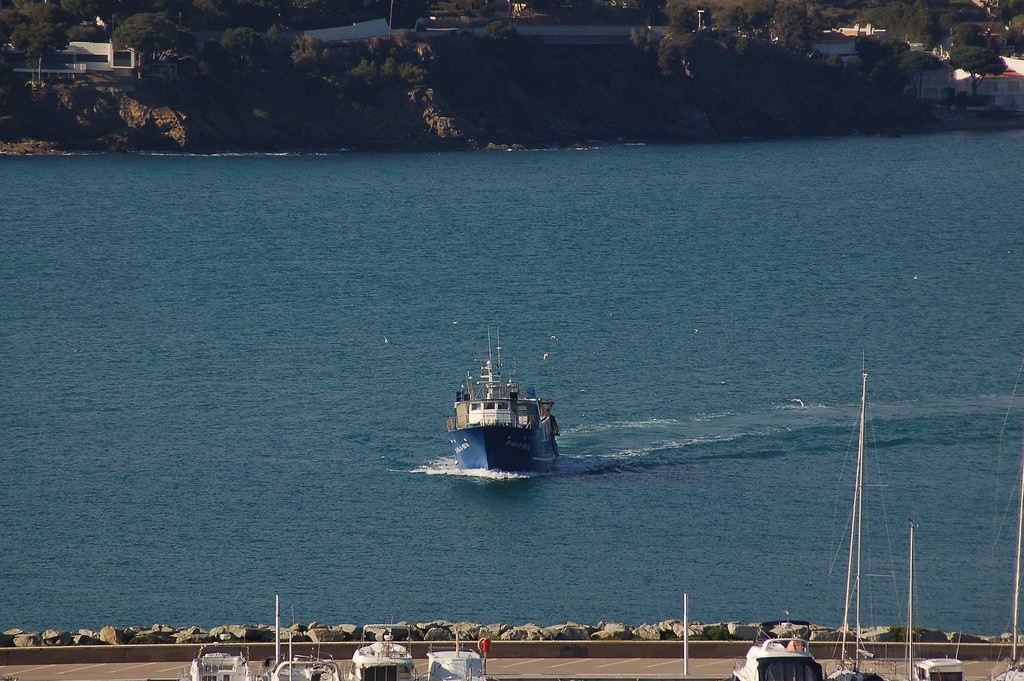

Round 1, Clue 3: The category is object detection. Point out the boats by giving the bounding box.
[255,594,341,681]
[342,623,418,681]
[176,643,255,681]
[415,630,492,681]
[725,366,1024,681]
[445,324,561,472]
